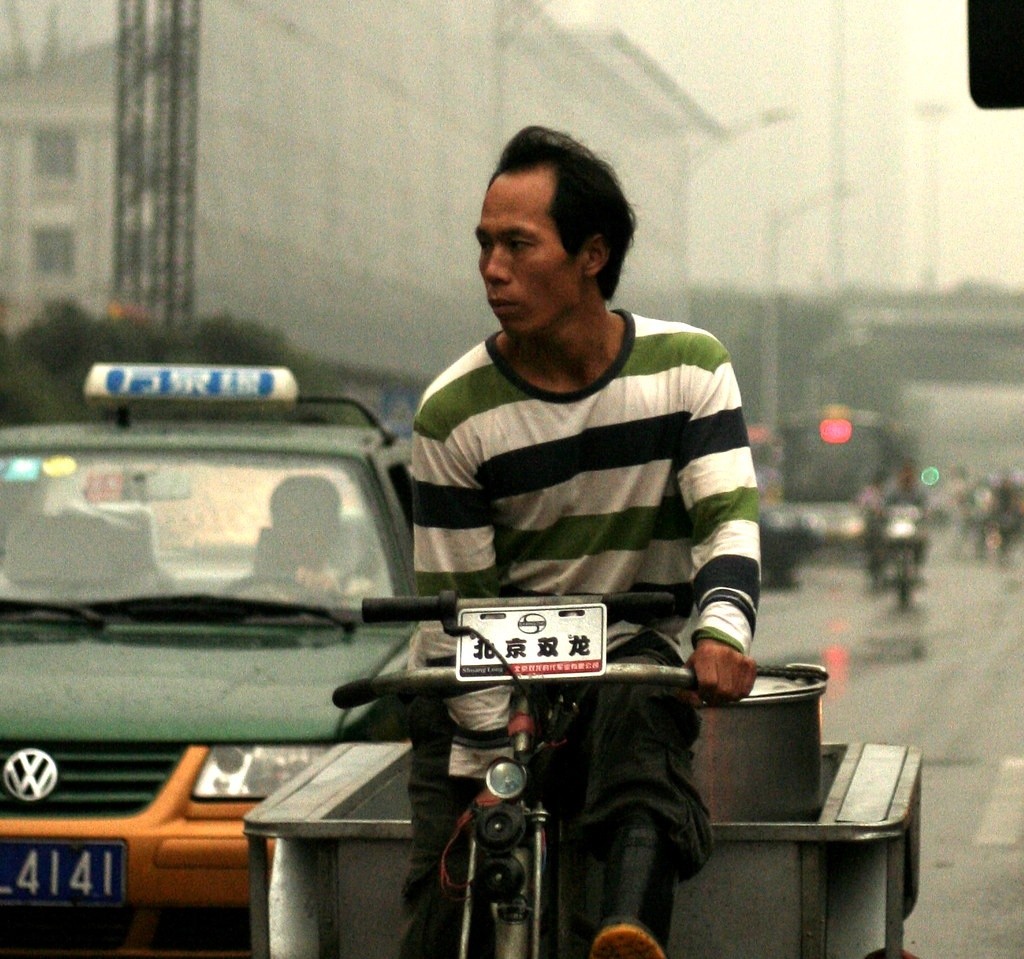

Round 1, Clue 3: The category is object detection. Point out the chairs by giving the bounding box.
[49,505,152,596]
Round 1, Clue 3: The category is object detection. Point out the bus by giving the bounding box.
[773,405,903,554]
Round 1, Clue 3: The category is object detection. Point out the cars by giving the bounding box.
[0,364,419,909]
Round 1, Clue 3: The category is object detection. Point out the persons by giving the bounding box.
[862,457,1024,596]
[236,476,379,602]
[396,126,761,959]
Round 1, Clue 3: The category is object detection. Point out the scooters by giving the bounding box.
[870,498,926,608]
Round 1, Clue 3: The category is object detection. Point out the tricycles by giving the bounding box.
[246,587,920,959]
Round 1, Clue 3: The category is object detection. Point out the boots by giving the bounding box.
[589,823,668,959]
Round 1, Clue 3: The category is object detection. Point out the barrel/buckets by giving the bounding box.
[691,663,829,823]
